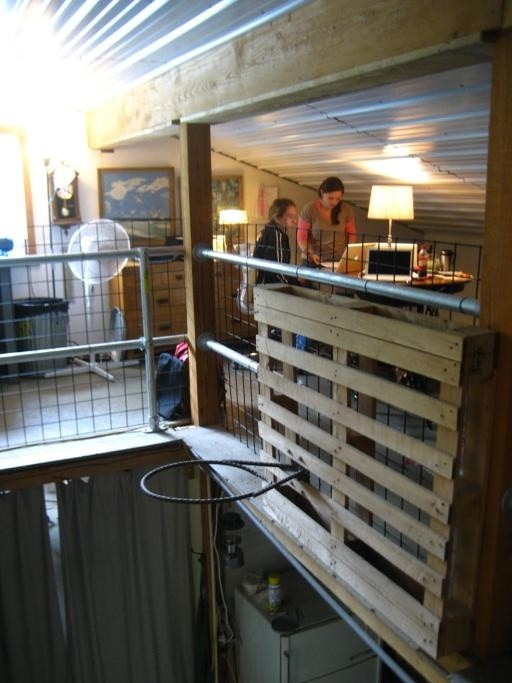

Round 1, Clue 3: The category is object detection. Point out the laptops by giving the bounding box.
[364,249,413,282]
[325,242,379,274]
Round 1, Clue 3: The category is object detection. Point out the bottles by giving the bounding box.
[439,249,455,271]
[418,244,429,279]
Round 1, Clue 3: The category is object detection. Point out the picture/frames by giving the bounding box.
[212,175,245,243]
[98,166,176,247]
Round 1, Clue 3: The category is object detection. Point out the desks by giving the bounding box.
[319,266,470,295]
[0,257,41,383]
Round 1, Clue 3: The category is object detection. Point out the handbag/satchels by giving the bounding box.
[156,352,226,421]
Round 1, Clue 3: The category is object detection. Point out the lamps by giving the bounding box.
[366,184,414,246]
[220,209,249,253]
[219,511,245,569]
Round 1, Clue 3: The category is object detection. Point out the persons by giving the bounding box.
[296,176,358,285]
[252,197,310,375]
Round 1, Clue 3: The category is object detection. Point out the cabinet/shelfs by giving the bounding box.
[108,257,257,359]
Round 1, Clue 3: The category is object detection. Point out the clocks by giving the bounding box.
[47,162,81,235]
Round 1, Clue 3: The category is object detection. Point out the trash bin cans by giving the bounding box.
[14,297,69,378]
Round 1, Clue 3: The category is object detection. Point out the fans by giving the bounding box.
[44,218,143,382]
